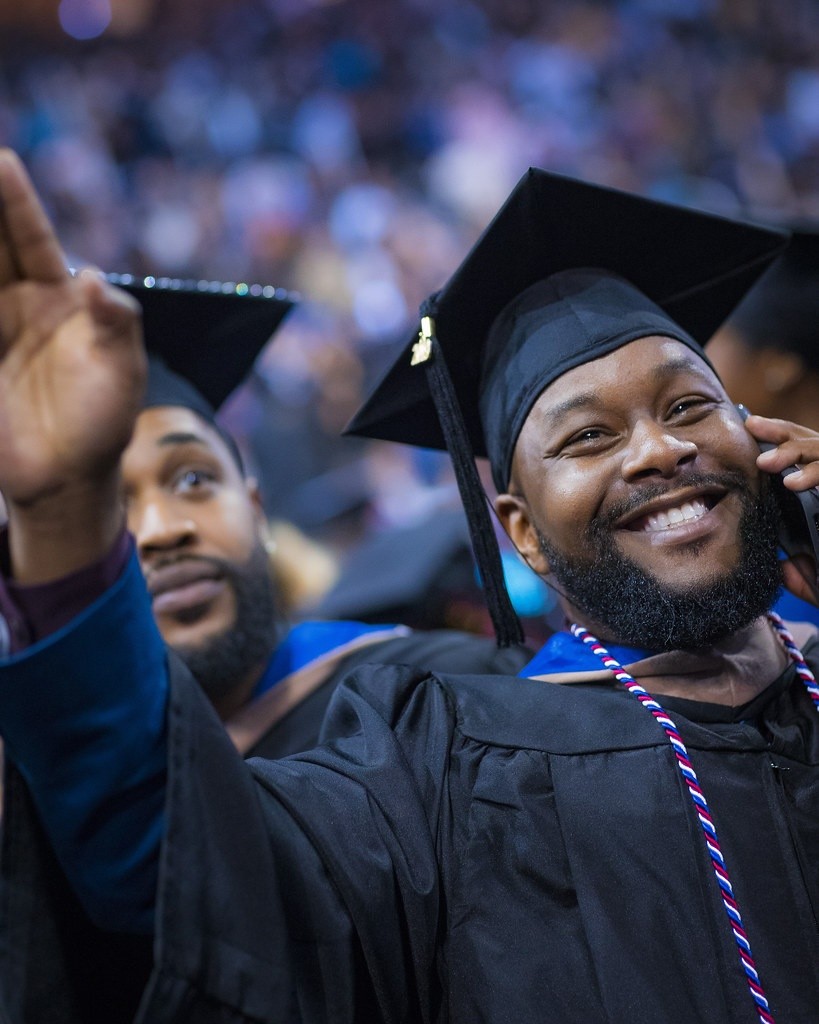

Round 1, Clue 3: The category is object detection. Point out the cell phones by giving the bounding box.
[733,401,819,598]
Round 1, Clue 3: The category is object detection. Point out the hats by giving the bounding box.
[70,270,302,422]
[342,167,793,651]
[304,494,473,632]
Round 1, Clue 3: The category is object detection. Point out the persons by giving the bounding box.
[272,467,550,649]
[0,146,819,1024]
[114,269,415,755]
[701,216,819,606]
